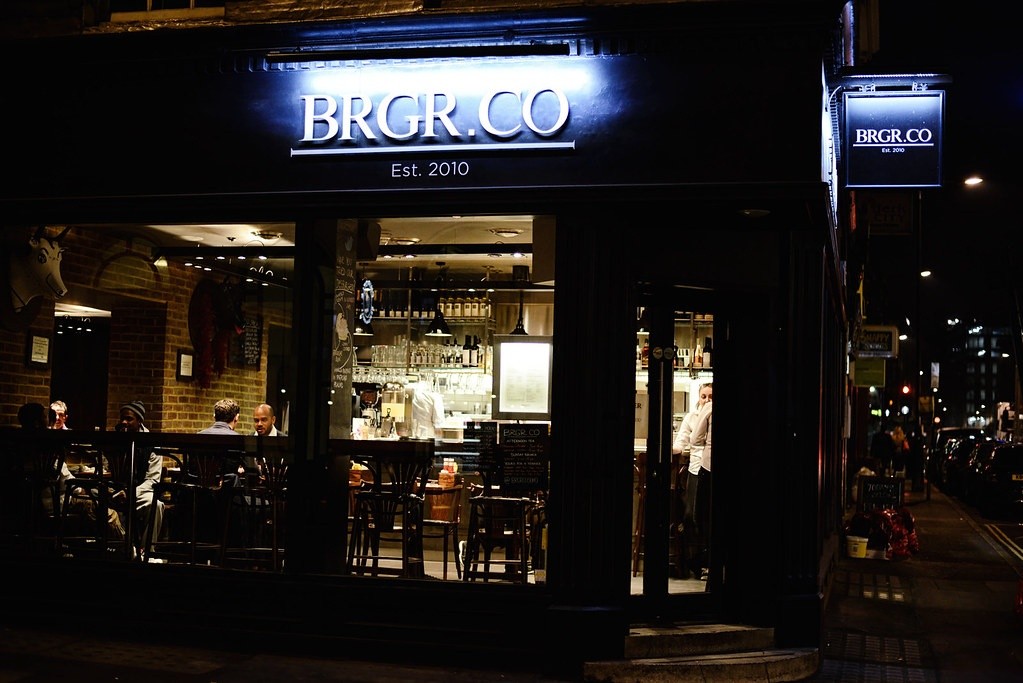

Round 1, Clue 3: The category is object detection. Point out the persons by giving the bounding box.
[673,381,712,579]
[245,404,288,480]
[16,403,125,540]
[49,401,107,473]
[188,399,257,474]
[689,402,712,581]
[405,370,444,438]
[871,423,910,474]
[102,401,164,550]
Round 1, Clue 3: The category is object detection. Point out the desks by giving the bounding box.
[364,480,462,494]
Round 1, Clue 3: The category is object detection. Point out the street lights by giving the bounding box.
[898,174,985,494]
[901,405,910,434]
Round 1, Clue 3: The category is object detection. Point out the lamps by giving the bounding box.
[425,293,453,337]
[489,228,519,237]
[352,262,374,336]
[506,291,529,334]
[391,237,420,245]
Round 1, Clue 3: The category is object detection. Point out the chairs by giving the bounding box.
[0,438,546,588]
[631,450,691,577]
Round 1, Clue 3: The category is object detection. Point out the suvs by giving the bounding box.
[936,427,986,458]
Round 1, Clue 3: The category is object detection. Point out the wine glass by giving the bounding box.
[352,346,358,366]
[352,368,408,384]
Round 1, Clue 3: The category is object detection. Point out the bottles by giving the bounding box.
[371,333,482,368]
[356,289,491,318]
[442,458,456,473]
[636,336,713,370]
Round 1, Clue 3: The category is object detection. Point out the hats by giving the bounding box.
[120,399,145,423]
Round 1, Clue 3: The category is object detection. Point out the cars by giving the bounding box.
[947,439,1023,510]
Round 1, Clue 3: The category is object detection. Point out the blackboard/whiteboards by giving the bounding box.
[331,350,354,426]
[460,422,498,473]
[499,424,550,497]
[227,312,262,372]
[857,476,904,515]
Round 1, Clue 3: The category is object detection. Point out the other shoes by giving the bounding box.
[668,567,691,580]
[701,567,709,581]
